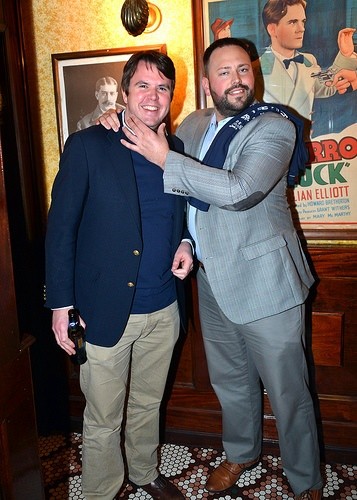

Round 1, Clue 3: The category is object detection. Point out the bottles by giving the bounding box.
[67,309,87,365]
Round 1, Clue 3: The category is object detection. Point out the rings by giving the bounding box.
[190,268,193,271]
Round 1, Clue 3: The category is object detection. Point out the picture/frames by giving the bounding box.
[191,0,357,239]
[52,43,172,154]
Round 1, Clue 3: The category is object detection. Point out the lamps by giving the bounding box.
[120,0,162,37]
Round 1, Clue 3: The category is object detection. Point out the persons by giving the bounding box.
[45,51,194,499]
[76,76,125,131]
[95,38,322,500]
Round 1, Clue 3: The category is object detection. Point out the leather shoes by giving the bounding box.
[296,488,319,500]
[141,475,186,500]
[206,456,260,492]
